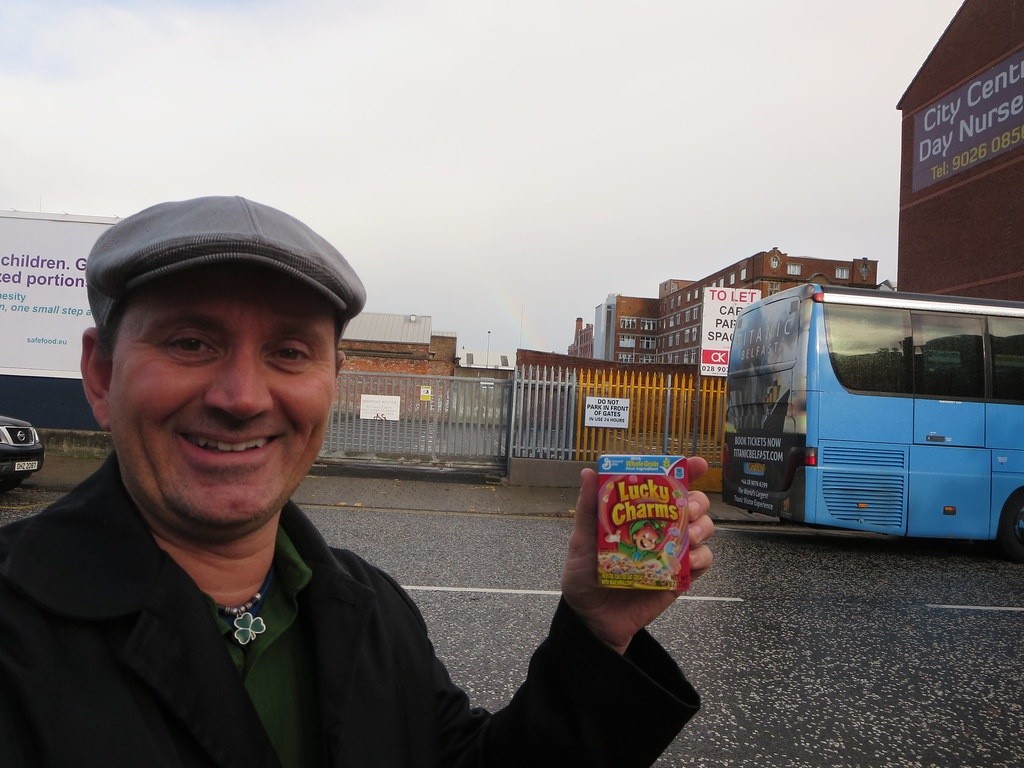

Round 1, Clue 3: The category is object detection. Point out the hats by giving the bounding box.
[84,195,366,343]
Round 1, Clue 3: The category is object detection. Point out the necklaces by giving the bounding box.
[213,567,274,645]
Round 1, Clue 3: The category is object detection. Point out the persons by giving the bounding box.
[0,196,715,768]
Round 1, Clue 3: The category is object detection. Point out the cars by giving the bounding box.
[0,414,44,491]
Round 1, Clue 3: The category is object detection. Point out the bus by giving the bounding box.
[721,283,1024,558]
